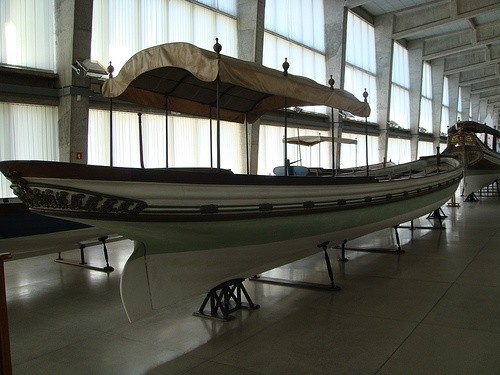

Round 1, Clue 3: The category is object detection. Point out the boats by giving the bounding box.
[418,119,499,203]
[0,33,466,324]
